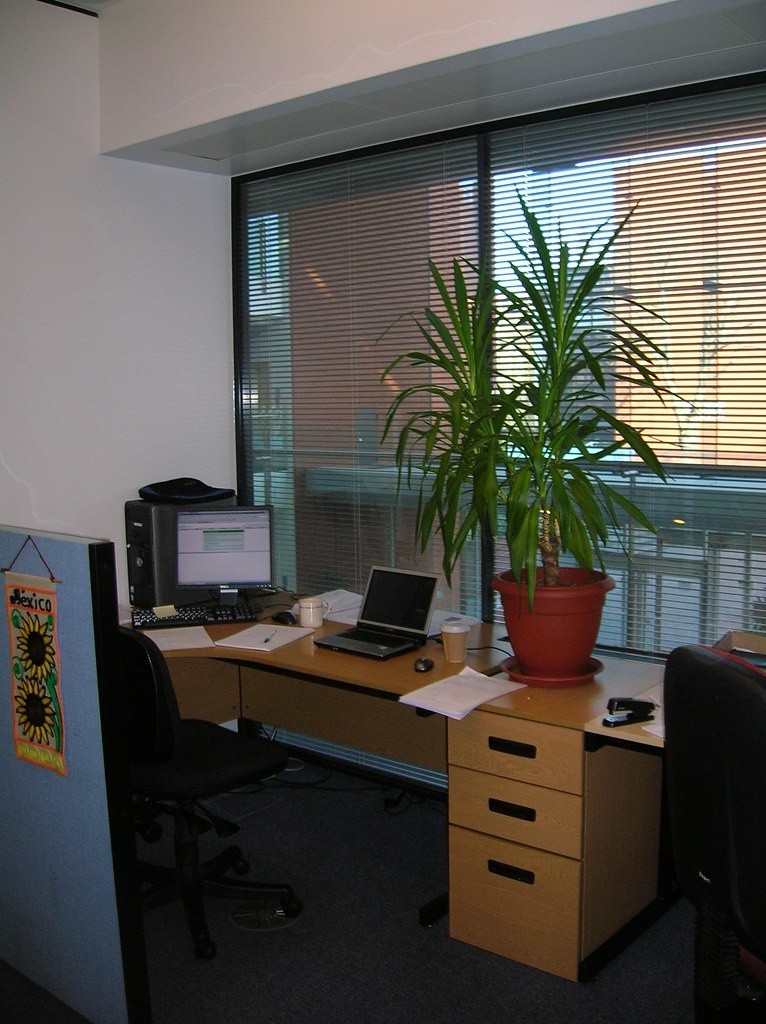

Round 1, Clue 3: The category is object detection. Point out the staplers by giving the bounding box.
[603,697,655,728]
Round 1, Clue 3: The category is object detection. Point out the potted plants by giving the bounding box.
[373,183,696,690]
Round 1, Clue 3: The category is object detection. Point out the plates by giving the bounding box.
[500,656,605,686]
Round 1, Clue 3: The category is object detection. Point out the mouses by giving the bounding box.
[272,611,297,625]
[415,657,434,673]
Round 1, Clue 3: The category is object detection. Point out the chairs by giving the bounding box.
[118,626,304,961]
[663,643,766,1024]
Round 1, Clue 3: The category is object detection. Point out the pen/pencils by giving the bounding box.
[265,630,278,644]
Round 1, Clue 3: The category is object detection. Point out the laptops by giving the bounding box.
[311,566,443,660]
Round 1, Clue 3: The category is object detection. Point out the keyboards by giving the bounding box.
[132,606,259,630]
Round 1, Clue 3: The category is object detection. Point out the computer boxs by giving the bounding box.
[125,490,247,611]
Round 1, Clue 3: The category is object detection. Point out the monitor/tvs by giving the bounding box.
[174,506,274,607]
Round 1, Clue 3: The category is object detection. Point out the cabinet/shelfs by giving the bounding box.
[122,590,666,985]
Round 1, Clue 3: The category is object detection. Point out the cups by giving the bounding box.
[298,598,330,628]
[441,621,470,663]
